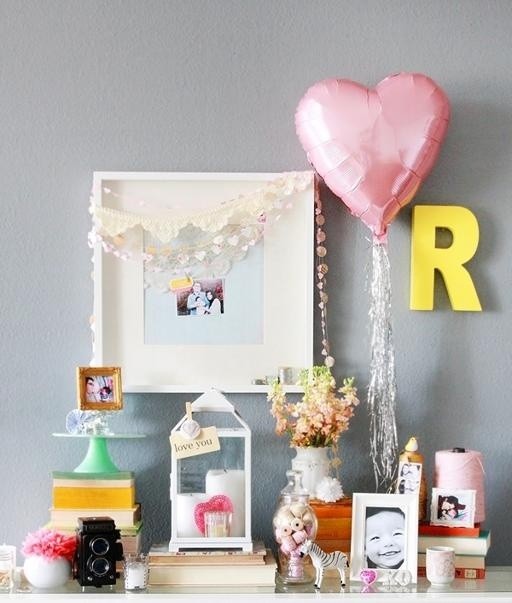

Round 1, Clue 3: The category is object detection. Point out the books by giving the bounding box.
[47,466,278,585]
[417,519,492,579]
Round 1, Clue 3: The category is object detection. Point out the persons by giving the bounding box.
[99,386,110,401]
[397,463,420,493]
[195,295,205,314]
[436,494,458,518]
[84,375,99,402]
[186,281,210,315]
[203,288,221,313]
[365,506,407,569]
[440,508,456,518]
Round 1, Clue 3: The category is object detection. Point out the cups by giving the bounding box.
[203,510,233,539]
[122,552,150,591]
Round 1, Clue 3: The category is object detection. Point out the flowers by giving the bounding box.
[20,527,77,566]
[266,365,361,450]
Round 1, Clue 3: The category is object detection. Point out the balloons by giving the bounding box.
[294,71,450,243]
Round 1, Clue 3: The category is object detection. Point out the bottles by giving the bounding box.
[271,467,321,586]
[397,436,428,521]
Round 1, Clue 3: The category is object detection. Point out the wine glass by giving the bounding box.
[425,546,456,593]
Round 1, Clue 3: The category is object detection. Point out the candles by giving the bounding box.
[176,468,246,538]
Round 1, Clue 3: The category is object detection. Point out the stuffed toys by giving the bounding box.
[299,536,349,588]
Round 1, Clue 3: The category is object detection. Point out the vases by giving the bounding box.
[291,447,332,500]
[22,557,71,588]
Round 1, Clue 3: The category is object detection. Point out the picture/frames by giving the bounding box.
[349,493,420,585]
[76,365,124,412]
[429,487,477,529]
[91,171,316,395]
